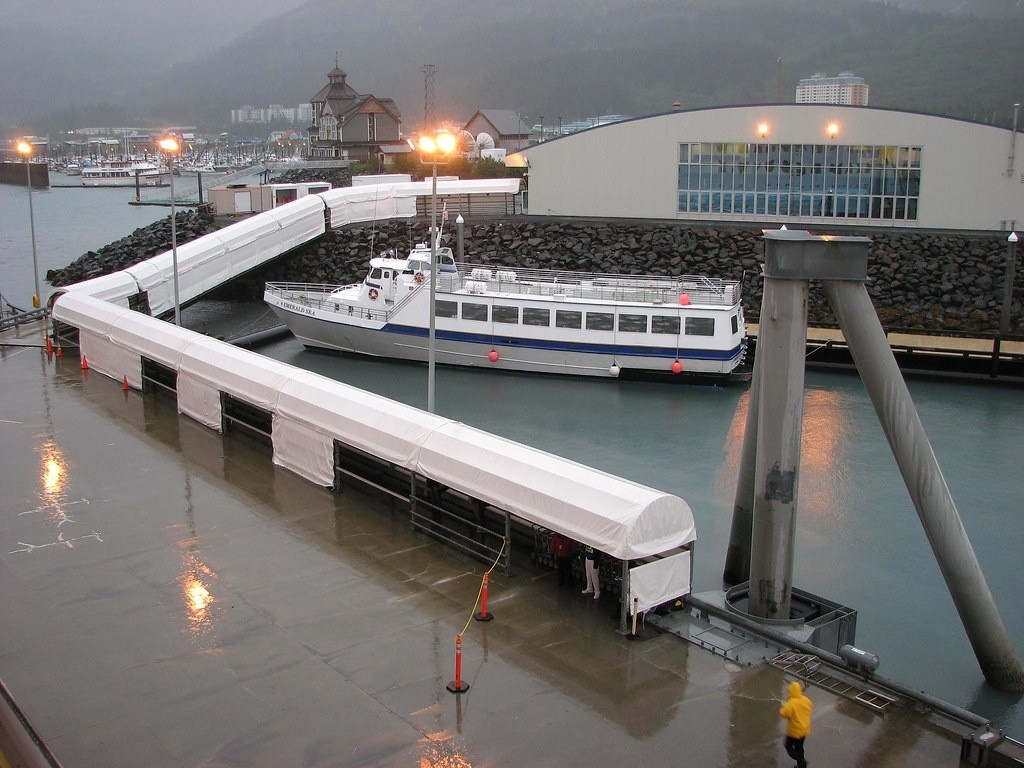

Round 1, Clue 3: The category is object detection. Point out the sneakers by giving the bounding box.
[582,589,593,593]
[594,592,600,599]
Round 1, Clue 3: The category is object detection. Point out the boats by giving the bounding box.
[264,228,749,381]
[3,150,302,188]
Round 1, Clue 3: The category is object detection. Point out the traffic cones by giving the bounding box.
[121,373,131,390]
[56,341,64,356]
[46,338,56,352]
[80,354,90,369]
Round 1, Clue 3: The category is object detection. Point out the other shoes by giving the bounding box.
[794,765,799,768]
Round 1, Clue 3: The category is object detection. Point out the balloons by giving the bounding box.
[609,366,620,375]
[489,352,499,362]
[672,362,682,373]
[679,294,690,305]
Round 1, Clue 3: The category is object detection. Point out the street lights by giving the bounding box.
[539,114,545,143]
[415,133,455,415]
[558,116,563,139]
[507,113,530,151]
[160,137,183,327]
[17,138,42,306]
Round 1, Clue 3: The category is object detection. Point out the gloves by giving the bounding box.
[593,568,599,574]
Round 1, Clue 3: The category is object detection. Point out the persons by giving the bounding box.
[780,681,813,768]
